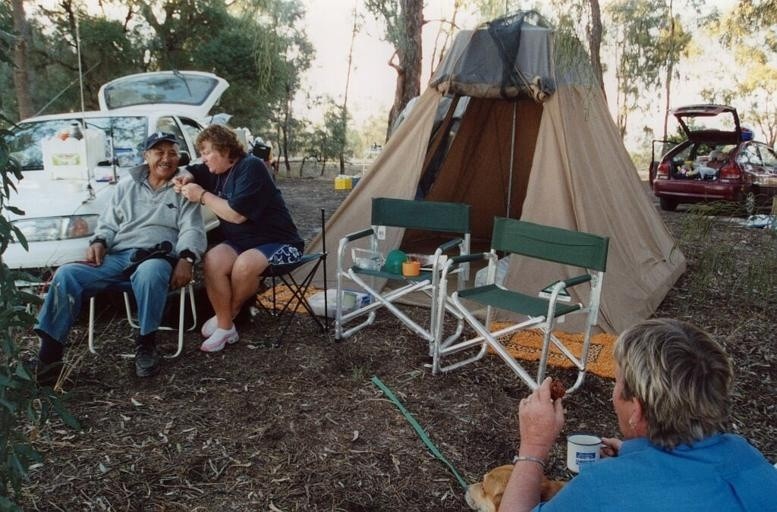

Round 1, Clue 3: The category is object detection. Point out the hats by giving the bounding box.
[145,131,180,151]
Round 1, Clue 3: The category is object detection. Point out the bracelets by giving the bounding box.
[512,455,546,468]
[200,190,207,206]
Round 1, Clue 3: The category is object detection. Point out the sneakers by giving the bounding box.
[201,314,219,339]
[135,333,159,377]
[23,357,63,384]
[200,323,239,352]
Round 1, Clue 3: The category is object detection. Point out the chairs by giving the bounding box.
[433,217,610,394]
[247,208,330,348]
[88,265,197,360]
[335,197,471,358]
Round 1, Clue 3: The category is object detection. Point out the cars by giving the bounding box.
[1,69,229,270]
[645,102,776,215]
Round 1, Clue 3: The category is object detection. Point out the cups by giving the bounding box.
[343,290,357,306]
[568,436,609,473]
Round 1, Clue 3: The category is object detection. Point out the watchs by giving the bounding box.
[182,257,193,263]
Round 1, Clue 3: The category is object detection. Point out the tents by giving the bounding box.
[283,9,686,335]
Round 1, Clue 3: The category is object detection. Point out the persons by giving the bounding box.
[32,132,207,387]
[172,124,306,353]
[495,318,777,512]
